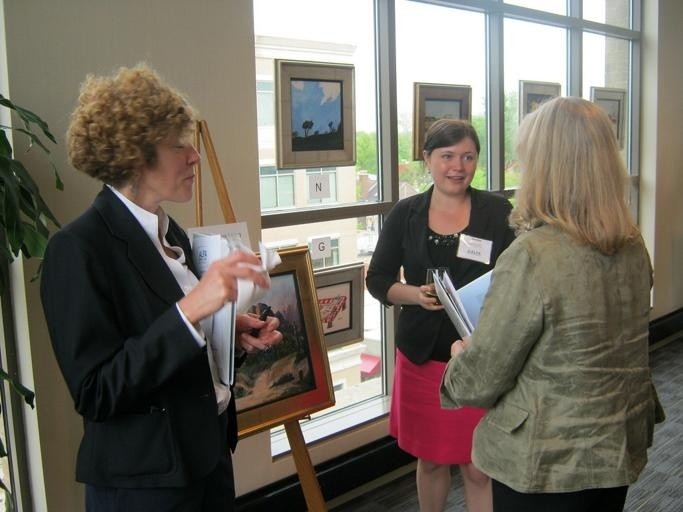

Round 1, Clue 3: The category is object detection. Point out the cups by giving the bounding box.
[424,267,451,303]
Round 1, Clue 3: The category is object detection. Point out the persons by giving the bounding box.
[438,97,666,512]
[365,118,515,512]
[41,60,285,512]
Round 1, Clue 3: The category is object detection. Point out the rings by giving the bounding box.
[264,344,273,354]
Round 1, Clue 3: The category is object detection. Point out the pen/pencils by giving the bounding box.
[235,306,271,368]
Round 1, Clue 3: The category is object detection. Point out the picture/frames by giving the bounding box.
[411,80,473,161]
[274,56,359,170]
[516,79,562,130]
[313,260,365,353]
[590,86,627,150]
[205,246,336,444]
